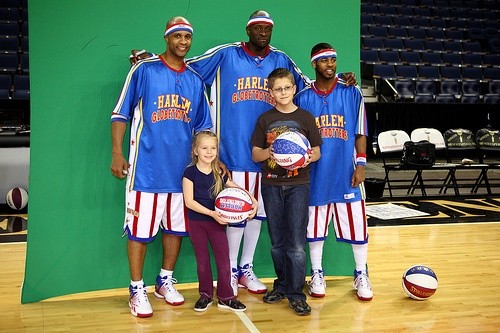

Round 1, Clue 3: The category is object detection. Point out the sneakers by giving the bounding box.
[263,289,288,303]
[194,295,213,311]
[237,265,267,294]
[154,275,185,305]
[354,272,373,301]
[128,284,153,317]
[218,299,246,311]
[230,267,238,296]
[307,270,325,297]
[289,298,311,316]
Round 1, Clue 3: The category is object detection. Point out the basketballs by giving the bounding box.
[6,186,29,210]
[215,188,252,224]
[273,130,312,171]
[402,265,438,301]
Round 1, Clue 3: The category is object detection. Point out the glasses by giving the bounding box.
[271,85,294,92]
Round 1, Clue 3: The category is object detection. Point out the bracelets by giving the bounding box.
[356,153,367,166]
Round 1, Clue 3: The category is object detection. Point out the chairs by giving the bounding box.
[0,0,29,146]
[360,0,500,199]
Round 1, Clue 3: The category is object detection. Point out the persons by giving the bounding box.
[250,67,323,316]
[293,43,374,301]
[182,129,257,312]
[111,16,231,317]
[128,11,359,298]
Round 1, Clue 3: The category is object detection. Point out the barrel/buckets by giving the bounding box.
[364,178,385,200]
